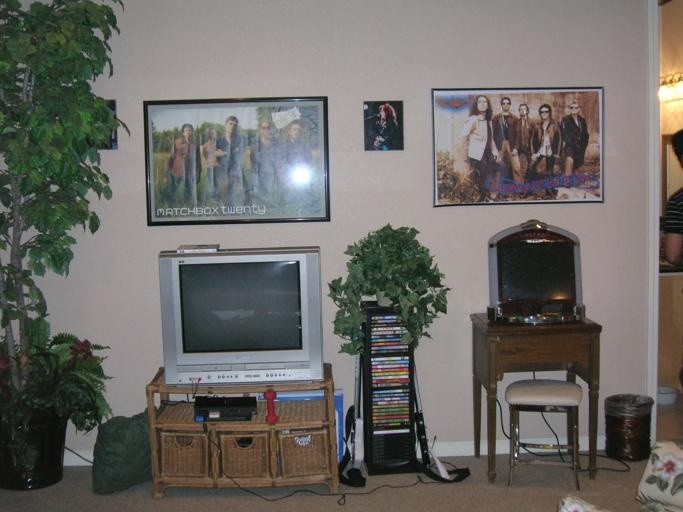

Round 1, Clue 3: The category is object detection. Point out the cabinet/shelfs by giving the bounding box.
[143,364,341,498]
[356,299,419,477]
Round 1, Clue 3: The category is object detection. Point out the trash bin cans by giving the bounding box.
[604,394,655,461]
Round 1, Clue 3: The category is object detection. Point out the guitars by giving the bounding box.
[414,363,432,473]
[349,353,364,464]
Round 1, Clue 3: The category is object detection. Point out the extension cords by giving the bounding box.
[520,444,569,454]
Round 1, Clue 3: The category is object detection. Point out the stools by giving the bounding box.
[503,379,586,489]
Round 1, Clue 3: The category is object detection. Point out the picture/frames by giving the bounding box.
[661,133,682,216]
[142,96,330,226]
[430,86,605,209]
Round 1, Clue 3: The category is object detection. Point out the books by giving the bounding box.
[368,312,413,435]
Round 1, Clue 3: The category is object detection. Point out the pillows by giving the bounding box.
[632,435,682,512]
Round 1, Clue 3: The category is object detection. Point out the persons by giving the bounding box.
[457,92,590,197]
[165,94,315,210]
[663,126,683,276]
[363,103,397,150]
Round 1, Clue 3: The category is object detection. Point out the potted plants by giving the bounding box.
[0,0,131,491]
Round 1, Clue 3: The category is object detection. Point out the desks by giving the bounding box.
[470,311,603,484]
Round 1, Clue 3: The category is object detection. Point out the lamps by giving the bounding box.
[657,70,683,102]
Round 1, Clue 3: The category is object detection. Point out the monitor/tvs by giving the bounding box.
[158,246,323,385]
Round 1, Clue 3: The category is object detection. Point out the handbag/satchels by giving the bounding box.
[92,407,161,496]
